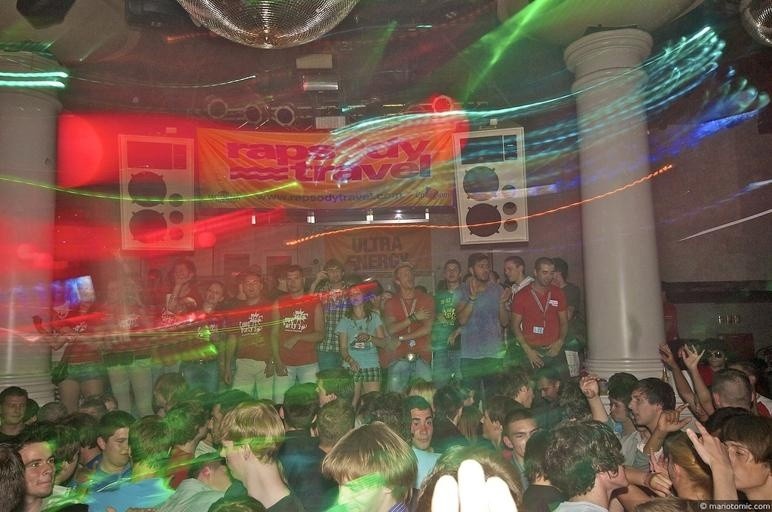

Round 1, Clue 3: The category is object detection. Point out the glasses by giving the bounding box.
[705,351,723,359]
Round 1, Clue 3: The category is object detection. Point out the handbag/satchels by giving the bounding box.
[50,361,69,385]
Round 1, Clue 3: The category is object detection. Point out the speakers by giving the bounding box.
[451,122,530,246]
[117,135,196,252]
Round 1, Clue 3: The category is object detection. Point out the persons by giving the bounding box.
[0,251,771,512]
